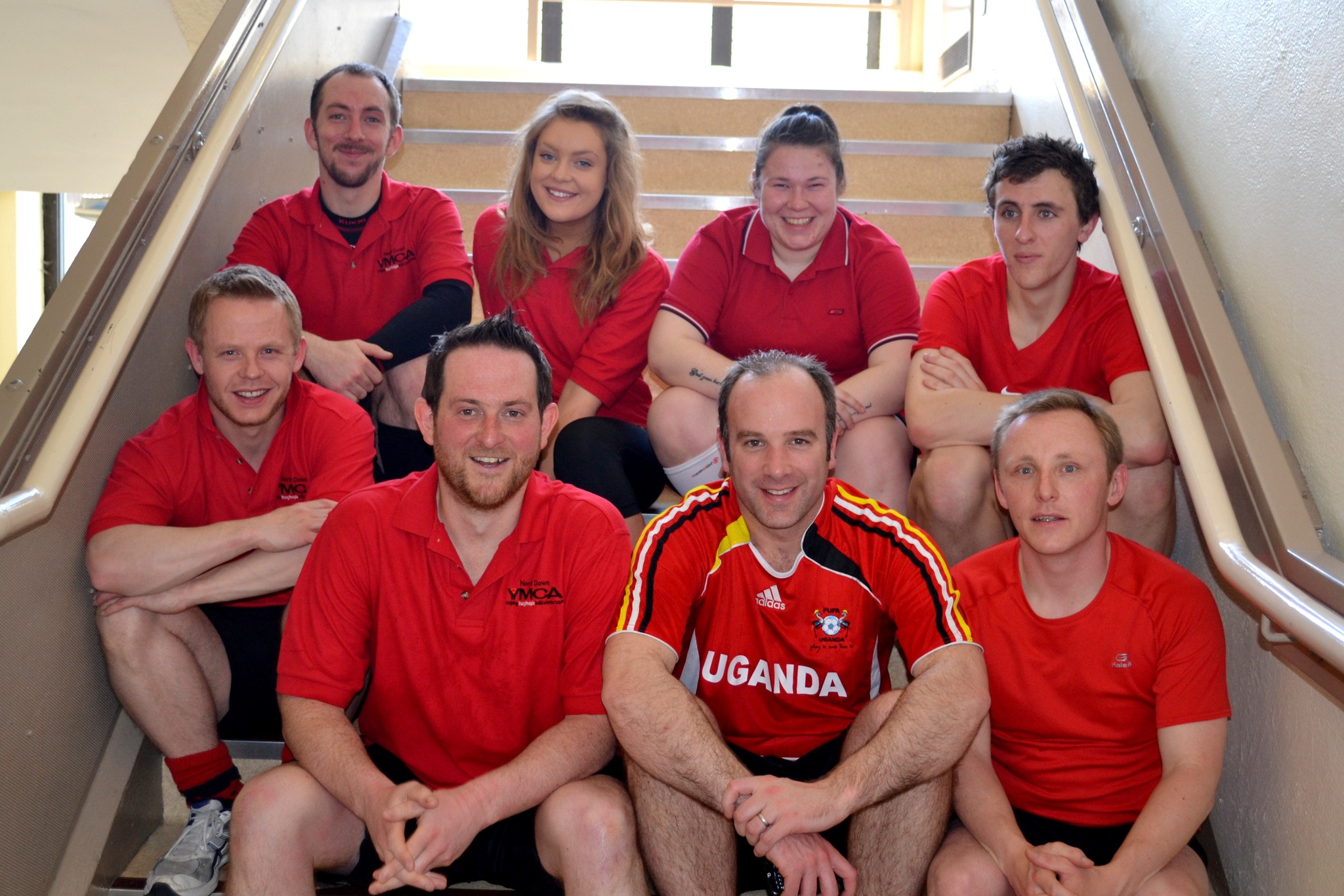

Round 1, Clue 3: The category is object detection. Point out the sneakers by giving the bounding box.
[143,798,233,896]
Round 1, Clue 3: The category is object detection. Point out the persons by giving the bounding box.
[644,102,924,525]
[216,62,474,483]
[925,389,1233,895]
[903,132,1173,557]
[81,263,378,896]
[599,348,994,896]
[473,86,673,556]
[222,303,649,896]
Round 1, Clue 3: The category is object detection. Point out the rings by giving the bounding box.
[757,813,770,827]
